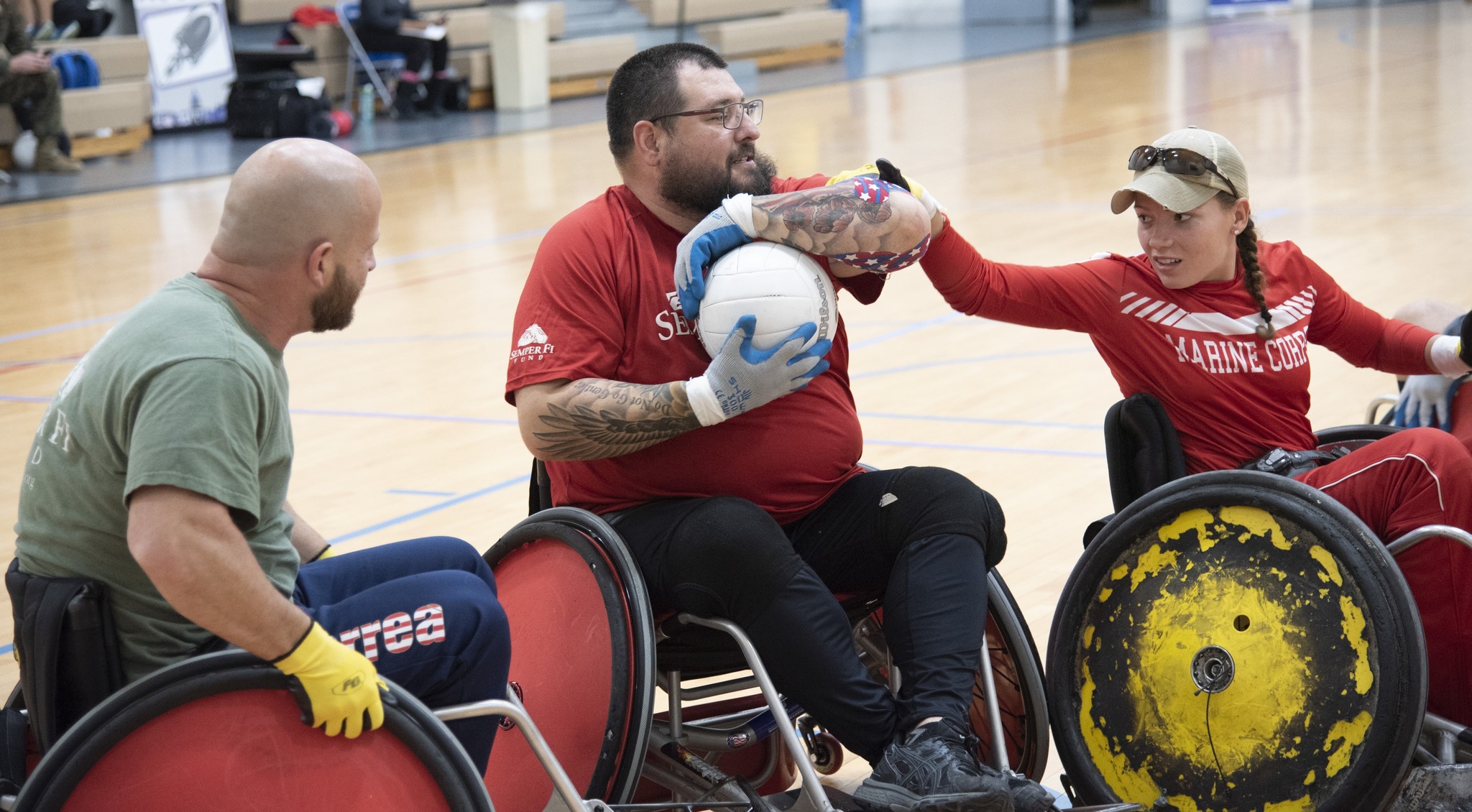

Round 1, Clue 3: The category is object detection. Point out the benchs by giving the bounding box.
[0,0,851,160]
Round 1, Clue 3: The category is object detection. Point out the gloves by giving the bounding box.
[1394,375,1468,434]
[270,619,397,739]
[826,157,937,223]
[673,192,758,322]
[687,315,831,426]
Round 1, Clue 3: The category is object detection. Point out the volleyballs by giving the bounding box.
[695,241,841,366]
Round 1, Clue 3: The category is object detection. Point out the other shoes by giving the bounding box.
[32,138,83,171]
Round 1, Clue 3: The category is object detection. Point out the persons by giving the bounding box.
[0,0,84,173]
[15,138,514,779]
[824,129,1472,722]
[506,42,1055,812]
[359,0,450,82]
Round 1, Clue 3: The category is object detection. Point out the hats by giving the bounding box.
[1111,125,1250,213]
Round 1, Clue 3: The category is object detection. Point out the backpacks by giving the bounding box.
[49,52,98,89]
[228,75,335,138]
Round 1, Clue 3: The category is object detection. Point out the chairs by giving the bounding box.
[335,2,407,117]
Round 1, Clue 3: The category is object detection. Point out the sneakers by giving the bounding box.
[852,716,1015,812]
[978,763,1056,812]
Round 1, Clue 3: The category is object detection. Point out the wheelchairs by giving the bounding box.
[0,558,493,812]
[482,456,1051,812]
[1045,374,1472,812]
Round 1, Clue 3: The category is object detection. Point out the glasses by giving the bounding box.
[1129,146,1240,200]
[649,99,764,130]
